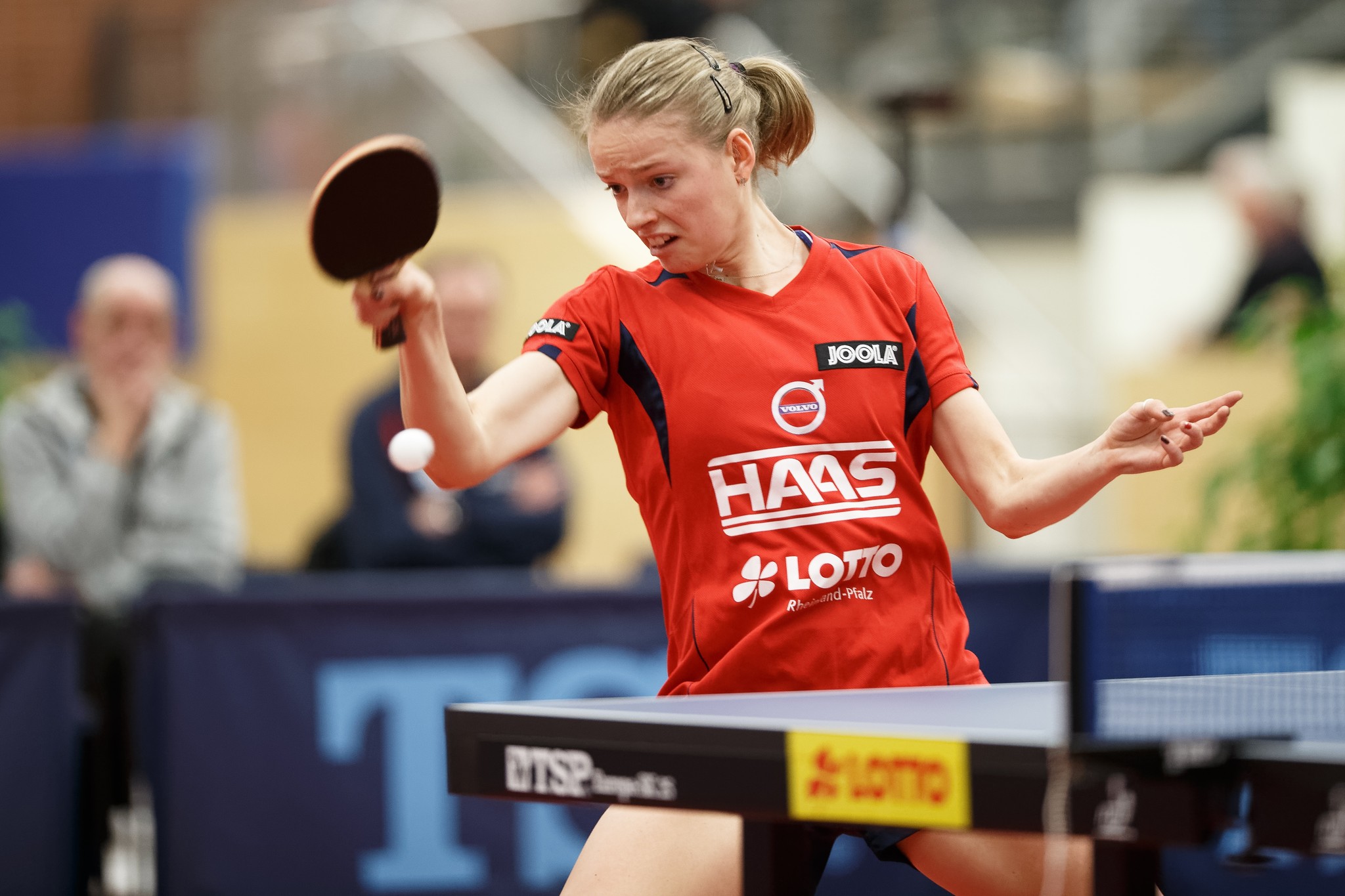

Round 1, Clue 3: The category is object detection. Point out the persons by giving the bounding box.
[1203,133,1326,350]
[303,254,570,566]
[2,254,247,598]
[354,37,1246,894]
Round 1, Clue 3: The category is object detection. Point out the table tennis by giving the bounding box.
[389,427,436,471]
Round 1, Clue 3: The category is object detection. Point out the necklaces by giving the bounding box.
[705,223,797,282]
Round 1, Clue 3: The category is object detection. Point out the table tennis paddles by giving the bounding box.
[308,135,441,349]
